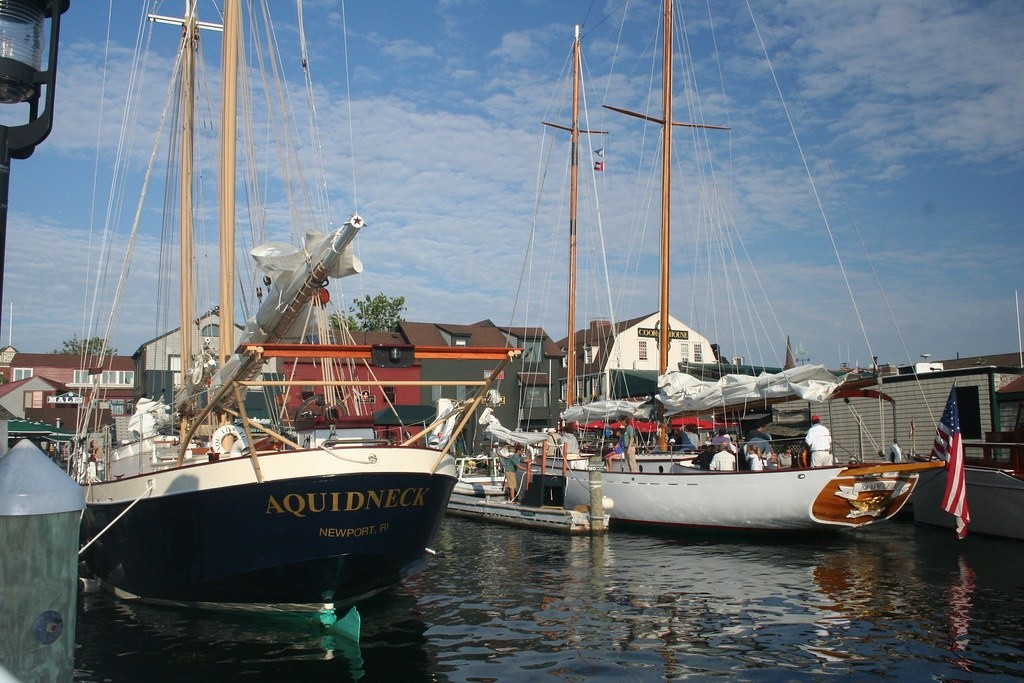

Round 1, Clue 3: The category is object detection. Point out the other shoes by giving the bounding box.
[511,498,518,502]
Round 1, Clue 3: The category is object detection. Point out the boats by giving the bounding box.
[907,415,1024,540]
[423,389,614,536]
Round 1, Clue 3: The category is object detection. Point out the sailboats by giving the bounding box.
[495,0,946,530]
[67,0,524,604]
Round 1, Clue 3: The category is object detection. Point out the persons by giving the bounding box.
[605,415,839,471]
[505,445,536,501]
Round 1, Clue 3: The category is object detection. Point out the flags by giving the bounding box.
[932,382,970,538]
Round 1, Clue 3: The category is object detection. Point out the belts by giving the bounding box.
[811,450,829,452]
[505,470,515,472]
[624,446,633,448]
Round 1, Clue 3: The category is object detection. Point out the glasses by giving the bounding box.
[519,450,524,452]
[787,448,791,450]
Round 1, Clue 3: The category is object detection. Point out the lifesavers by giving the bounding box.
[210,423,249,460]
[885,443,902,462]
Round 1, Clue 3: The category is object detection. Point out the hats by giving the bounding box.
[564,426,572,433]
[558,427,565,431]
[812,416,819,421]
